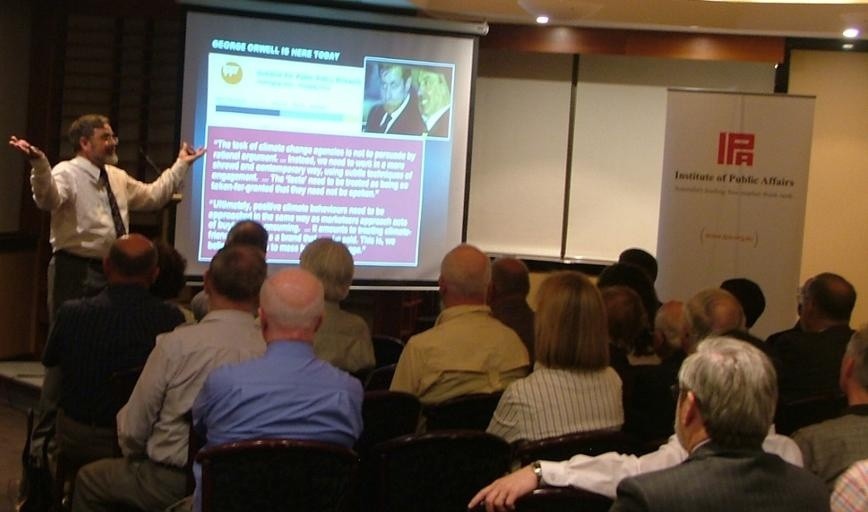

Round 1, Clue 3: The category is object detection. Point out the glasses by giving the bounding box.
[103,136,119,146]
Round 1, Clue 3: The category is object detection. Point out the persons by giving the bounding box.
[388,244,868,510]
[6,112,207,330]
[16,219,378,511]
[416,67,450,138]
[365,61,427,136]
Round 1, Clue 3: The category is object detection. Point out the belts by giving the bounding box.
[68,417,115,429]
[150,460,194,473]
[54,249,102,266]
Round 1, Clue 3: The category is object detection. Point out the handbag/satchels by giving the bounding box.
[17,406,56,512]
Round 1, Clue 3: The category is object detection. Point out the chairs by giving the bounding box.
[191,437,353,512]
[520,431,644,477]
[18,332,867,469]
[367,428,507,510]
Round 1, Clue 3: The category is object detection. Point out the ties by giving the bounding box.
[99,170,128,240]
